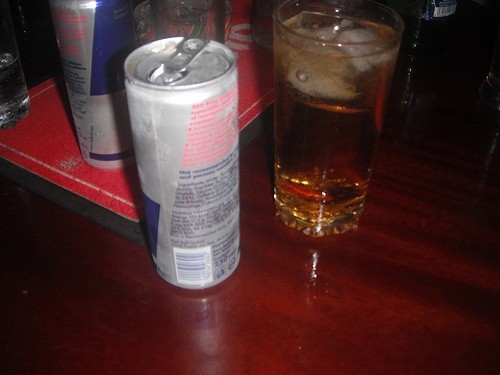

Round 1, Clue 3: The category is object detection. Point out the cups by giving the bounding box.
[267,2,402,241]
[134,1,224,46]
[1,0,30,129]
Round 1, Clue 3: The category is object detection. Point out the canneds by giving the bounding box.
[124,35,240,289]
[44,0,143,168]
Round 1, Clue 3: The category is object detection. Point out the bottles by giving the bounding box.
[391,0,463,39]
